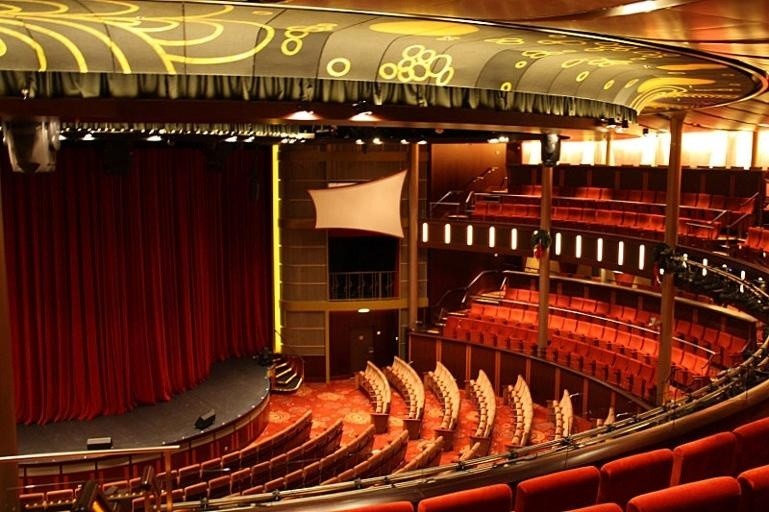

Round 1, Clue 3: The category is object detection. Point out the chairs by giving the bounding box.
[19,163,769,509]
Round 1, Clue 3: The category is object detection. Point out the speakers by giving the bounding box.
[194,408,216,430]
[541,134,562,167]
[86,437,113,451]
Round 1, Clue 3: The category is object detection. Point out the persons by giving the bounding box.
[196,497,217,511]
[508,450,519,460]
[559,438,570,446]
[75,484,83,500]
[456,461,467,471]
[384,477,392,484]
[271,491,282,501]
[606,424,616,432]
[629,253,769,425]
[355,480,364,490]
